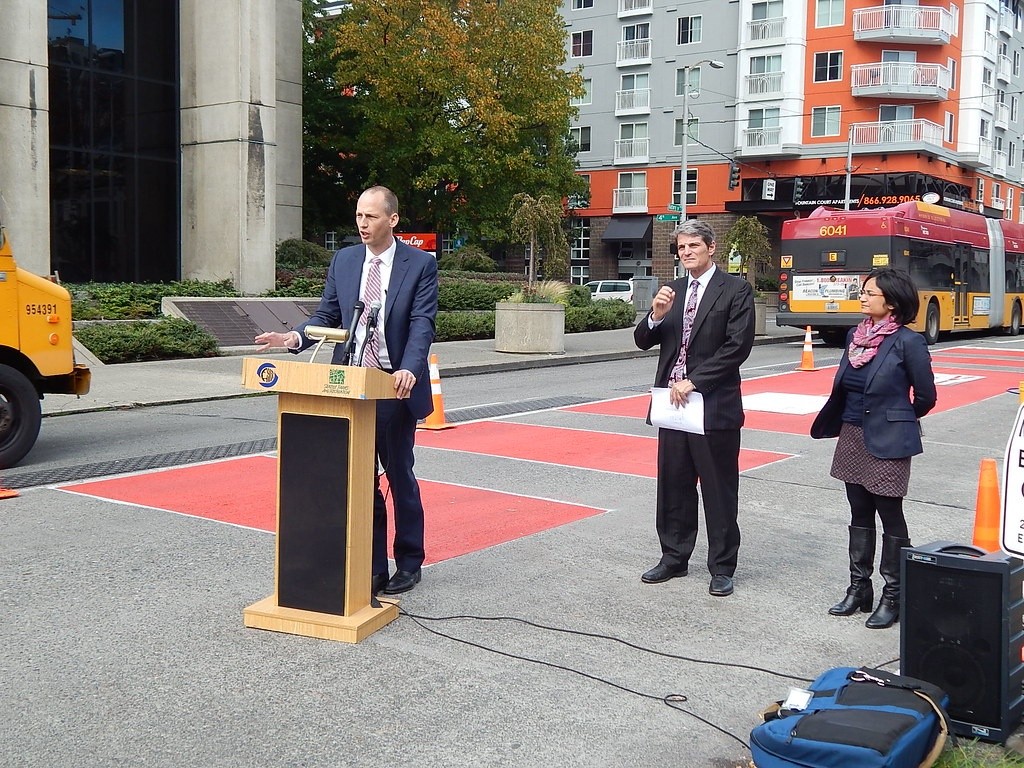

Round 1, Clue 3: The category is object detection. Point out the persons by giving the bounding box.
[634,220,756,596]
[848,279,858,300]
[810,267,937,629]
[255,186,437,595]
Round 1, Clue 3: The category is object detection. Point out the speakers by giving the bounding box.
[900,541,1024,745]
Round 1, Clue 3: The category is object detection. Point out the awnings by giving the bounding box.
[602,214,653,243]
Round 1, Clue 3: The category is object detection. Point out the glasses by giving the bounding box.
[860,290,884,296]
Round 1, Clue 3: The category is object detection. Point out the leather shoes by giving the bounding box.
[641,561,688,583]
[384,569,421,593]
[372,572,389,594]
[709,575,734,595]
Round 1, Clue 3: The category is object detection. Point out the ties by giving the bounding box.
[359,258,382,367]
[667,280,700,388]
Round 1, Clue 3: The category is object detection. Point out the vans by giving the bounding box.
[583,280,633,305]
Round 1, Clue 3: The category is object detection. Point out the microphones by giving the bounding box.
[343,301,365,353]
[366,300,382,343]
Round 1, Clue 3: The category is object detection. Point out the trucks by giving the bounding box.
[0,223,91,470]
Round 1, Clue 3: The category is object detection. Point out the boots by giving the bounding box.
[828,525,876,615]
[866,534,911,628]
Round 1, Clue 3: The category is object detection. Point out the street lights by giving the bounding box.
[678,59,724,278]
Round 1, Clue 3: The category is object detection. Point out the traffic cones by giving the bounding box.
[973,458,1001,554]
[415,353,457,430]
[794,325,822,372]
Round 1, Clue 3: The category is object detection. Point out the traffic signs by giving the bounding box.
[763,180,776,200]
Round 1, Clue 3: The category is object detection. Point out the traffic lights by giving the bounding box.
[728,163,741,191]
[793,178,805,204]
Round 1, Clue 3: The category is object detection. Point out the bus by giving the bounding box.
[776,200,1024,345]
